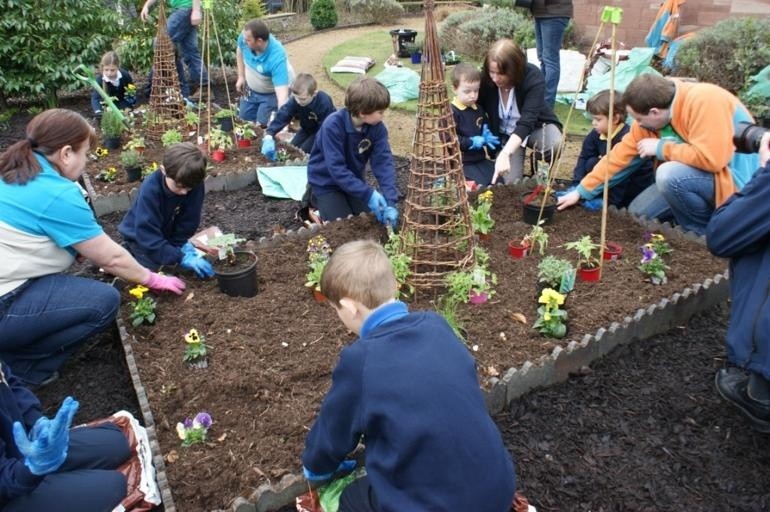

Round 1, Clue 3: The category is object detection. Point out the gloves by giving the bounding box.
[302,459,358,482]
[553,186,574,197]
[260,137,280,161]
[583,199,603,209]
[181,251,216,280]
[13,396,80,476]
[367,190,399,227]
[142,268,186,296]
[468,124,499,149]
[181,243,196,254]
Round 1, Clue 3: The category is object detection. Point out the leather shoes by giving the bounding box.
[714,366,770,433]
[187,87,216,101]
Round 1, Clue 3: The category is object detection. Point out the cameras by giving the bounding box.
[732,120,770,155]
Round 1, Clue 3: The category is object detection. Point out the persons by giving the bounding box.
[260,72,337,161]
[0,108,186,389]
[81,143,214,279]
[140,0,215,101]
[301,237,537,510]
[234,18,296,138]
[301,77,403,232]
[91,52,137,118]
[0,358,130,511]
[514,0,577,111]
[437,61,502,183]
[704,125,769,431]
[480,40,563,187]
[562,86,656,209]
[557,72,761,238]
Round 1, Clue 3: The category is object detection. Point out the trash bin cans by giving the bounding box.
[389,28,417,59]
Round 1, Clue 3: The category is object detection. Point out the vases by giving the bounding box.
[478,234,492,244]
[311,286,328,302]
[521,192,558,225]
[604,240,622,260]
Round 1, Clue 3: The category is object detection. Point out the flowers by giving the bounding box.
[472,191,496,234]
[304,234,333,287]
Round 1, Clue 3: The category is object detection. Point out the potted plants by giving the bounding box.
[188,112,198,131]
[536,254,562,294]
[215,110,234,132]
[124,83,136,102]
[209,136,231,160]
[559,260,576,293]
[210,233,259,299]
[136,138,144,153]
[509,218,547,258]
[235,126,251,148]
[122,150,141,182]
[561,235,609,282]
[469,264,488,304]
[101,112,122,149]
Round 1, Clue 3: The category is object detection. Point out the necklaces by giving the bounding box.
[496,86,513,93]
